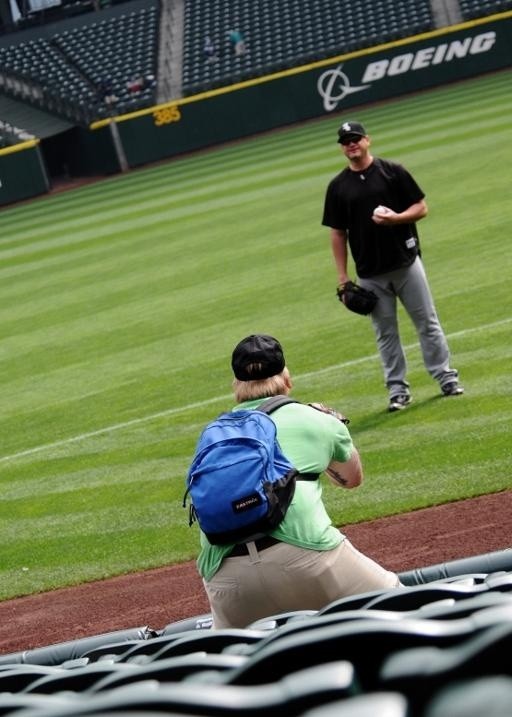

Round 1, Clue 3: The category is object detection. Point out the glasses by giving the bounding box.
[340,136,365,145]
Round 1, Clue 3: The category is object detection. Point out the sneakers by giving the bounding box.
[440,383,466,395]
[386,392,413,412]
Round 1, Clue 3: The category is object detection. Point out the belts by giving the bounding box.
[216,536,284,559]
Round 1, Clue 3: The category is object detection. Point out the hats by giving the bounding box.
[229,334,289,381]
[335,120,368,141]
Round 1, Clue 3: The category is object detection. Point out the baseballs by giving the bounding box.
[373,207,385,215]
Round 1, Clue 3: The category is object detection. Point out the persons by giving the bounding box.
[228,29,248,57]
[202,39,218,65]
[322,118,464,410]
[177,333,409,632]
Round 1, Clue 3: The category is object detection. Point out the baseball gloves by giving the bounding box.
[336,281,378,315]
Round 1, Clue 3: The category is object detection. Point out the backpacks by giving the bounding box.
[181,395,301,547]
